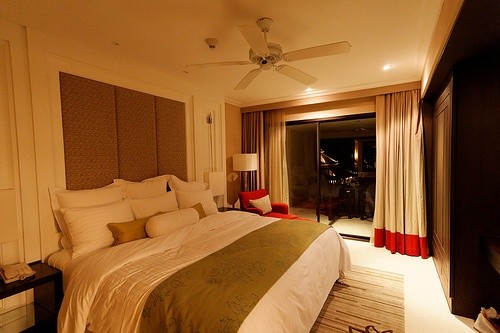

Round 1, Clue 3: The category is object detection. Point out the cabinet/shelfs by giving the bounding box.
[432,50,500,315]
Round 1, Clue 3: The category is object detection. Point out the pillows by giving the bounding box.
[249,195,272,214]
[49,174,218,259]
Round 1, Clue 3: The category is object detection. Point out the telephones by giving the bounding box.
[0,261,36,284]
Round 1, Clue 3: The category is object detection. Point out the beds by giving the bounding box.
[48,211,342,333]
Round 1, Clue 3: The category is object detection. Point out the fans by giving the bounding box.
[185,19,352,90]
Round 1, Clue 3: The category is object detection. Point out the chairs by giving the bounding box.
[238,189,288,217]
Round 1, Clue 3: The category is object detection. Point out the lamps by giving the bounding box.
[233,154,257,191]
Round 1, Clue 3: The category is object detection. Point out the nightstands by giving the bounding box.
[0,260,63,325]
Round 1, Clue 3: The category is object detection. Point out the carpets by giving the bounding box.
[310,265,405,333]
